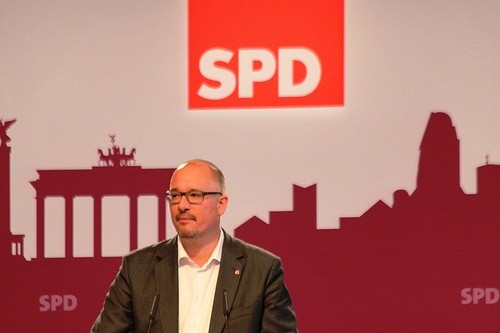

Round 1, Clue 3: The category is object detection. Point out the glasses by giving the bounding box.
[166,190,222,204]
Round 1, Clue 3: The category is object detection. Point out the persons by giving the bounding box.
[88,158,299,333]
